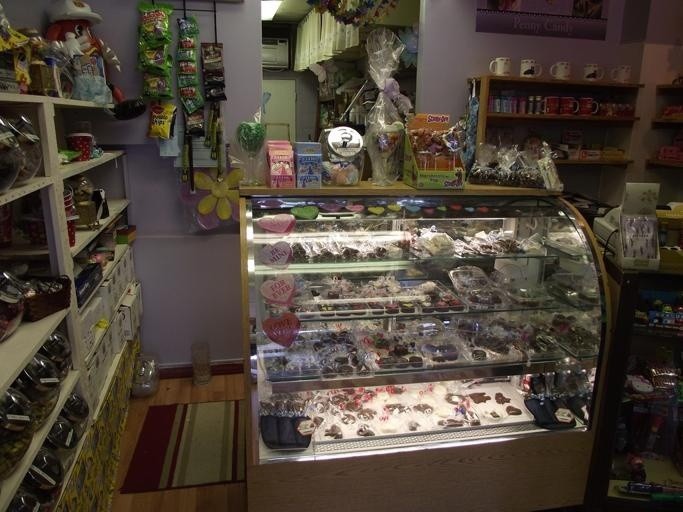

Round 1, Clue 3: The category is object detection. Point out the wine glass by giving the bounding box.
[235,121,265,187]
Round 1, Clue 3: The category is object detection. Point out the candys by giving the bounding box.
[410,128,458,172]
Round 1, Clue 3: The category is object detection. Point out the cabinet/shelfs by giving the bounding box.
[464,72,646,179]
[0,92,143,512]
[644,83,683,170]
[238,182,612,512]
[593,225,683,499]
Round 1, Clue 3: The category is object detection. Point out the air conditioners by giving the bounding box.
[262,37,290,71]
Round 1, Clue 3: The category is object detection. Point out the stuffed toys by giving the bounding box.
[43,0,146,119]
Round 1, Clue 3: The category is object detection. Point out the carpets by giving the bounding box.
[117,397,247,495]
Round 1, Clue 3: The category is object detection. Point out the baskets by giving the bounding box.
[14,273,71,322]
[19,185,80,246]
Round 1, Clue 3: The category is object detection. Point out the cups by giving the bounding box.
[611,65,633,82]
[550,62,571,80]
[489,57,512,77]
[541,95,600,116]
[583,63,604,82]
[520,59,542,78]
[27,189,76,249]
[191,341,210,384]
